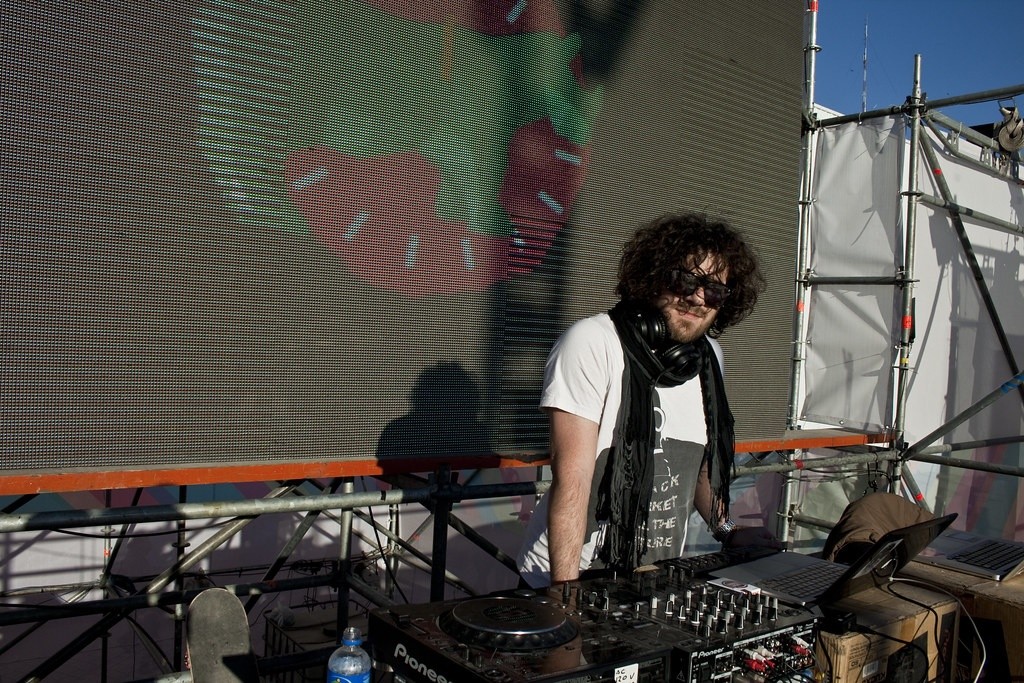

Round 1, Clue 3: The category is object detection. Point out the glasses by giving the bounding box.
[660,267,733,309]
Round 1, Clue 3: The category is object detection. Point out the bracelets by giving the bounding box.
[712,518,736,542]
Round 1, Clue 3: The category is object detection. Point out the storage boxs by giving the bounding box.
[900,561,1024,683]
[824,582,960,683]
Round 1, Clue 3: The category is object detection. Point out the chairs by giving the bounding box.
[184,584,258,683]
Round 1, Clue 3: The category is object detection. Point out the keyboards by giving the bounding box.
[666,547,778,573]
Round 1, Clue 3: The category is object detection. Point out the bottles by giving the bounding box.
[327,627,372,683]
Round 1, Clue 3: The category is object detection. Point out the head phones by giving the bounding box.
[616,297,698,377]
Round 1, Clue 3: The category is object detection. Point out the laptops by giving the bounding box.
[711,513,959,611]
[913,526,1024,582]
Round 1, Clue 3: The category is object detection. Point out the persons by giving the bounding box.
[513,207,782,583]
